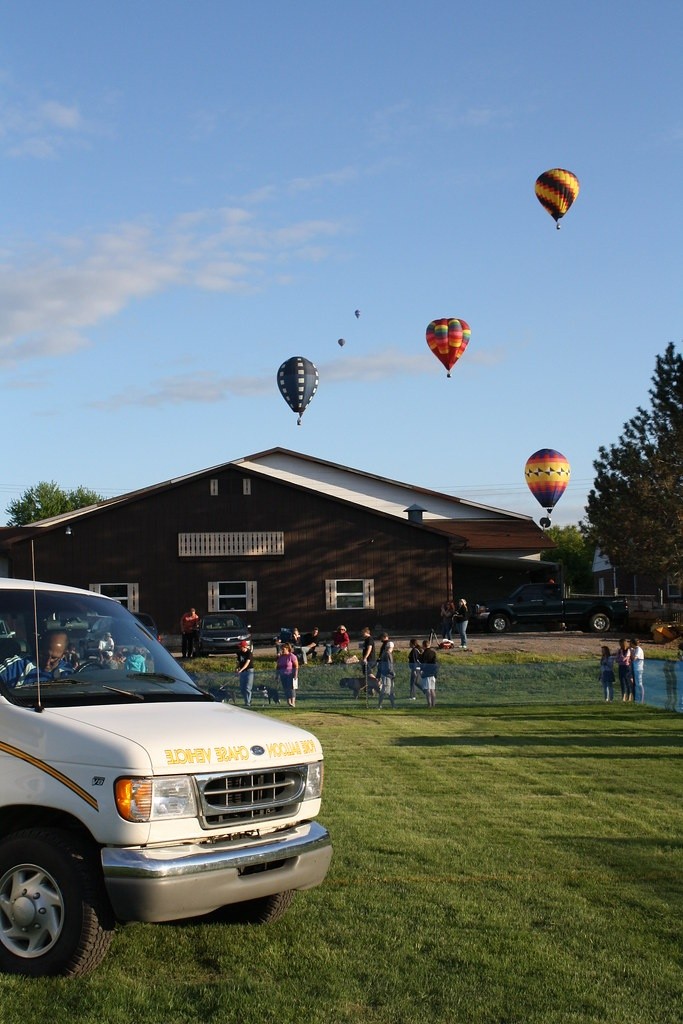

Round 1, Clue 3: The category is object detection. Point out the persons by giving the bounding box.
[377,641,396,709]
[0,630,74,689]
[374,632,393,696]
[362,627,383,695]
[616,637,645,702]
[180,608,199,658]
[600,646,614,702]
[322,625,350,664]
[275,642,299,707]
[272,626,320,664]
[452,599,469,648]
[408,639,427,700]
[236,641,254,706]
[98,632,146,673]
[441,600,455,640]
[419,640,436,707]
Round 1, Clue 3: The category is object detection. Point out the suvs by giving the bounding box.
[0,577,334,980]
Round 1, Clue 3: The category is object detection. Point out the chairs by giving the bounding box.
[0,638,30,665]
[226,619,233,627]
[207,623,212,628]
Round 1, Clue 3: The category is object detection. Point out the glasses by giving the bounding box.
[339,628,344,631]
[41,655,65,665]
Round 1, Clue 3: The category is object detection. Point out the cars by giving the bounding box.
[0,620,16,639]
[191,614,252,657]
[78,612,161,660]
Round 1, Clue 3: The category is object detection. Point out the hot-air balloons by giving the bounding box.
[277,356,319,426]
[355,310,361,319]
[426,318,471,378]
[338,339,345,347]
[525,448,571,521]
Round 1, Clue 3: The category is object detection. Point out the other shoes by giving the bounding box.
[458,645,467,648]
[408,697,416,701]
[326,660,333,664]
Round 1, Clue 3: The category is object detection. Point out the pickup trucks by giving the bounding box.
[486,583,630,634]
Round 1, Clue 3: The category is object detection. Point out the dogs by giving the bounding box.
[203,685,237,708]
[339,673,384,699]
[255,685,281,704]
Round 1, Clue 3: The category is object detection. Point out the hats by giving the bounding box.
[236,640,248,647]
[386,641,395,648]
